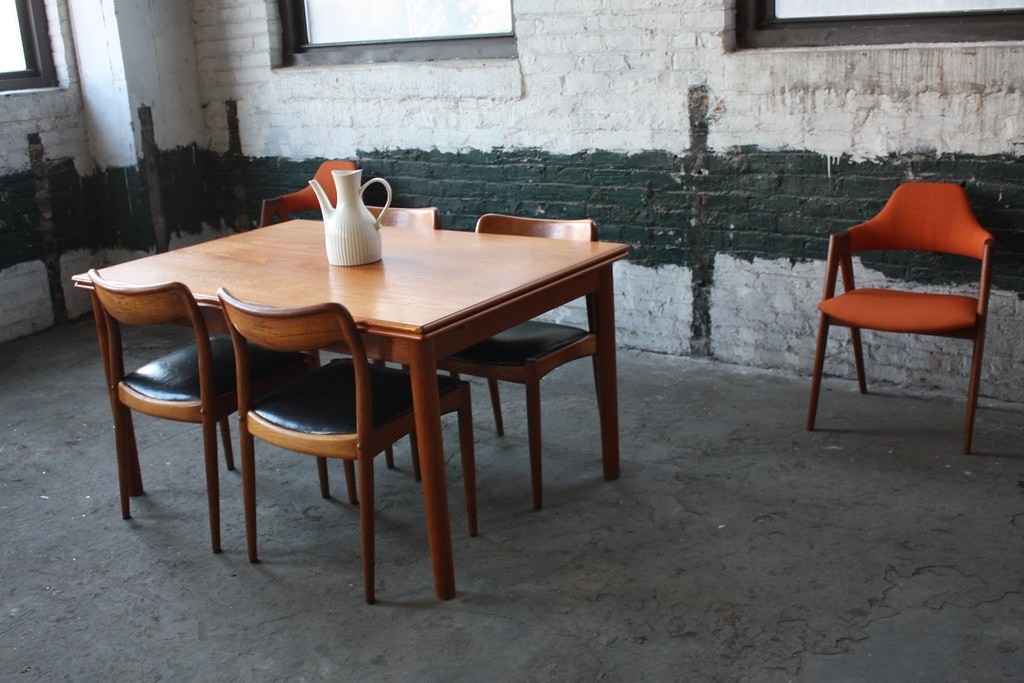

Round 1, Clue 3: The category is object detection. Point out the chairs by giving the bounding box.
[219,286,478,606]
[402,211,603,511]
[363,205,440,469]
[805,181,993,457]
[86,268,330,554]
[260,160,359,230]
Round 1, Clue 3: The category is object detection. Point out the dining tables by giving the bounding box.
[70,216,632,601]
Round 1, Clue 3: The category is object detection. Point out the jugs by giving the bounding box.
[307,168,392,266]
